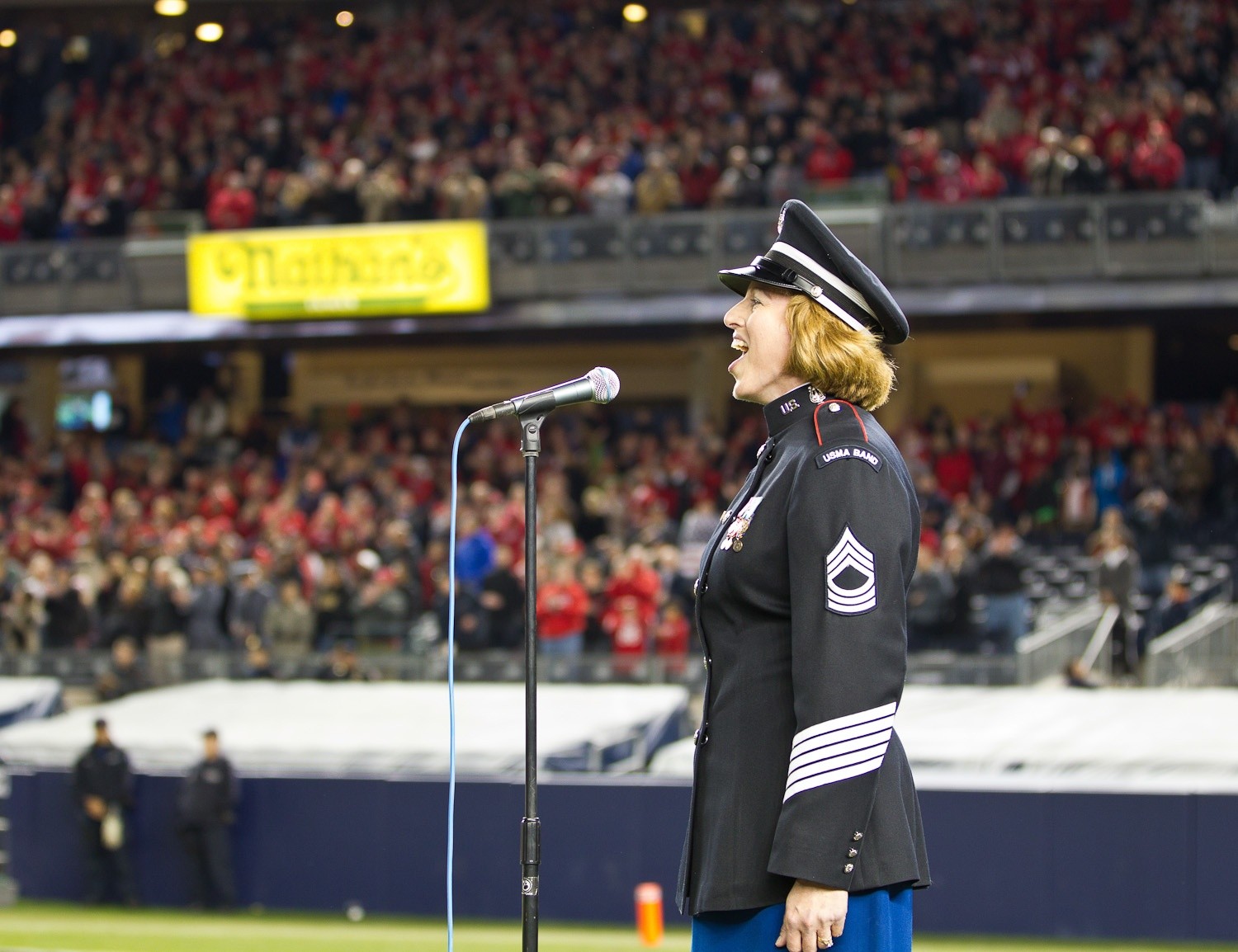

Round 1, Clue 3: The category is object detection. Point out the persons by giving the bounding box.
[181,730,236,909]
[74,720,141,907]
[1,380,770,711]
[675,201,932,952]
[0,0,1236,239]
[895,408,1238,653]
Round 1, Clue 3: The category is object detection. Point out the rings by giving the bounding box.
[818,936,833,947]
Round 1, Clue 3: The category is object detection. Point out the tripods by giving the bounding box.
[515,398,555,951]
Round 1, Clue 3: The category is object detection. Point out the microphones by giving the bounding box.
[468,366,620,426]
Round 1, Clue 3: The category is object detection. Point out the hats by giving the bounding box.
[721,200,909,347]
